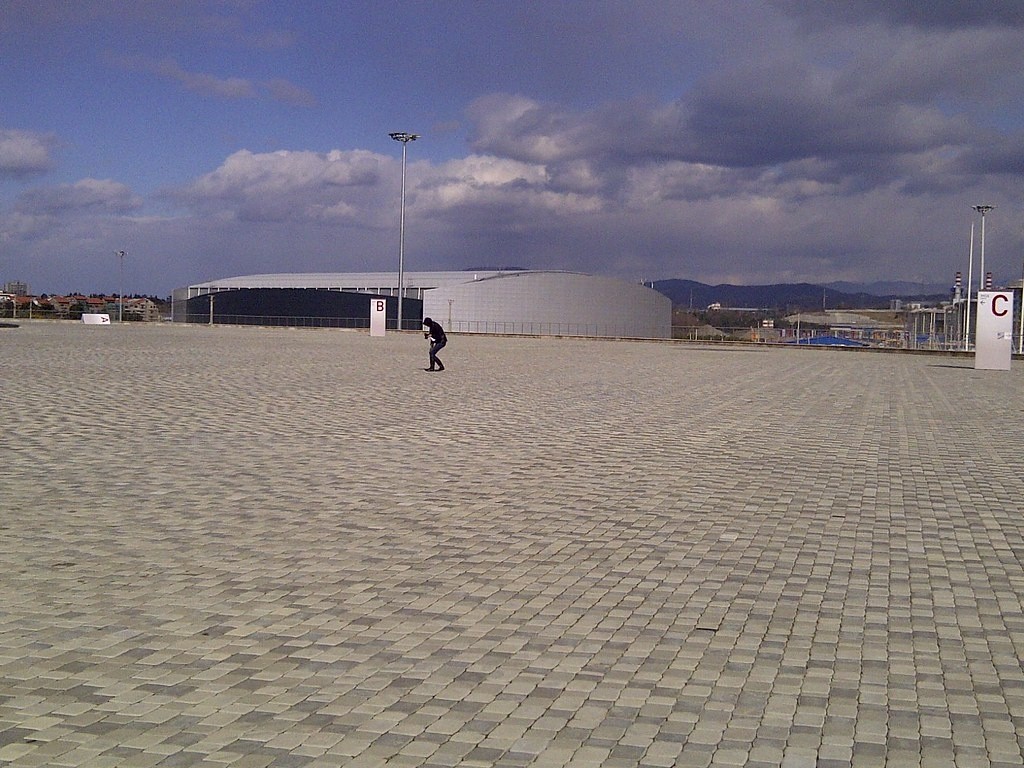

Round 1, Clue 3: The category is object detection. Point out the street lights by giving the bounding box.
[111,249,126,322]
[388,131,423,330]
[970,205,996,289]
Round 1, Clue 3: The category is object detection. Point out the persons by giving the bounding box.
[422,317,448,372]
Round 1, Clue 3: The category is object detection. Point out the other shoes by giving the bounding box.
[438,366,445,371]
[425,367,435,371]
